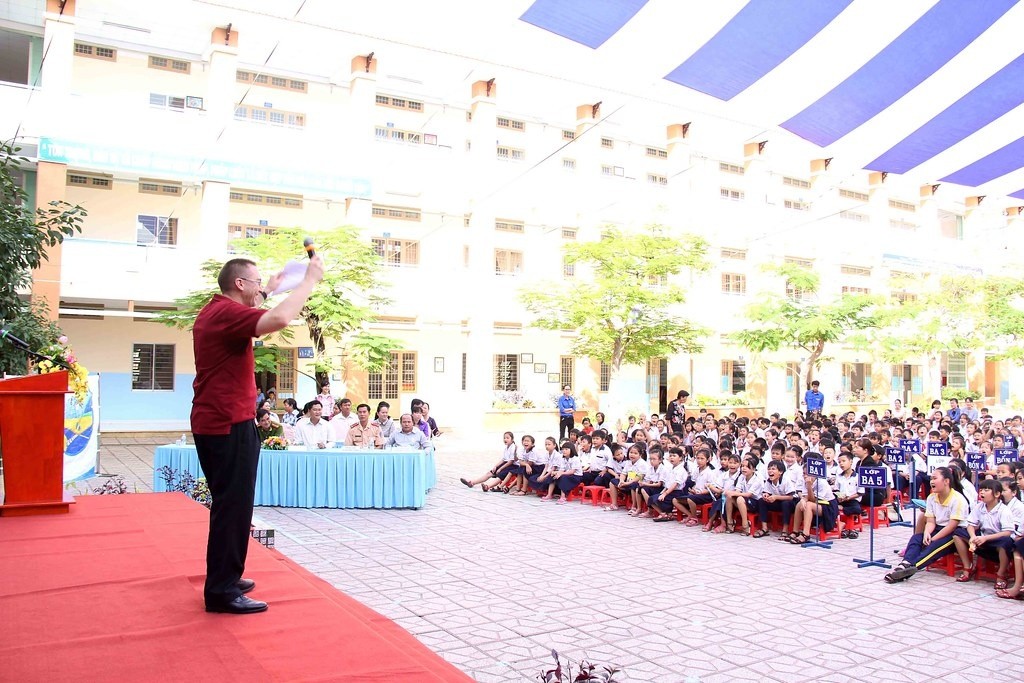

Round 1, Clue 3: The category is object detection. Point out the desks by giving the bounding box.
[153,438,437,511]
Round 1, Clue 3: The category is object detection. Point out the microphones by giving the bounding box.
[0,329,30,348]
[303,235,315,259]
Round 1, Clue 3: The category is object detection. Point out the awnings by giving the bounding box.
[517,0,1024,200]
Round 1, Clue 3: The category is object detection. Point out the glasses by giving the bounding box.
[241,277,262,287]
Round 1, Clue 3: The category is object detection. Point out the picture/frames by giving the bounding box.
[548,373,560,383]
[521,353,533,363]
[534,363,546,374]
[434,357,444,373]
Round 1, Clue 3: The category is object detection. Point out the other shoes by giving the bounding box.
[686,518,698,526]
[711,525,726,533]
[702,524,712,532]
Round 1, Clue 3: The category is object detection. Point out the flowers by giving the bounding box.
[261,436,290,450]
[31,336,90,406]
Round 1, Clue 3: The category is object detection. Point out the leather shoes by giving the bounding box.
[238,577,255,591]
[206,594,268,613]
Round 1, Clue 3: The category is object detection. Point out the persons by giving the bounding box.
[256,380,441,455]
[666,390,690,435]
[801,381,824,414]
[461,397,1024,599]
[558,384,577,438]
[189,254,323,614]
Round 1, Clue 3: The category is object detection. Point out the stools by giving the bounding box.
[499,473,1024,589]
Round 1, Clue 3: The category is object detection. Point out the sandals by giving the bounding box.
[490,486,503,492]
[995,589,1020,599]
[727,519,736,533]
[460,477,473,487]
[510,489,652,518]
[841,528,852,537]
[753,530,770,537]
[790,533,810,544]
[849,530,859,538]
[653,513,673,521]
[503,487,509,493]
[957,564,976,580]
[778,532,788,540]
[741,520,751,536]
[994,573,1008,589]
[481,483,489,492]
[681,516,694,523]
[885,562,917,583]
[784,531,803,541]
